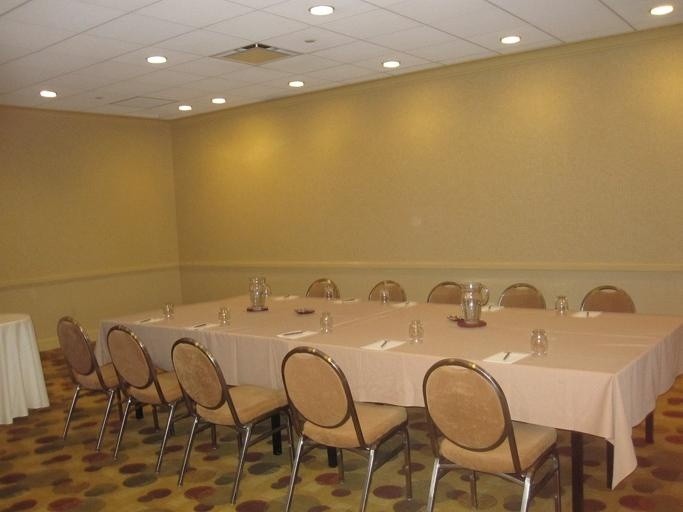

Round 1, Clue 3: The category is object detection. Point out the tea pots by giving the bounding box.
[247,276,271,307]
[460,282,489,324]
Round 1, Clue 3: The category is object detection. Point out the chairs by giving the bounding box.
[421,357,562,512]
[106,324,217,474]
[56,316,161,453]
[280,346,414,512]
[427,281,465,304]
[306,277,340,298]
[171,337,298,506]
[498,284,547,308]
[367,280,407,301]
[579,284,637,316]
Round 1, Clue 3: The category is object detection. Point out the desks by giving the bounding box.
[0,311,51,429]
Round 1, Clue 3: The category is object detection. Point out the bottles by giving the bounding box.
[218,307,232,327]
[554,295,570,317]
[380,289,392,305]
[410,319,424,346]
[530,328,548,360]
[161,302,175,321]
[325,285,334,301]
[320,311,333,336]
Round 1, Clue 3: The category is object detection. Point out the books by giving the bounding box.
[182,321,220,332]
[130,316,165,326]
[272,294,299,301]
[275,328,320,340]
[390,301,416,309]
[482,351,531,364]
[480,304,503,311]
[327,296,360,305]
[568,309,603,319]
[359,337,407,352]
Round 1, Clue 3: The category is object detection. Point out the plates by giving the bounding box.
[294,309,314,314]
